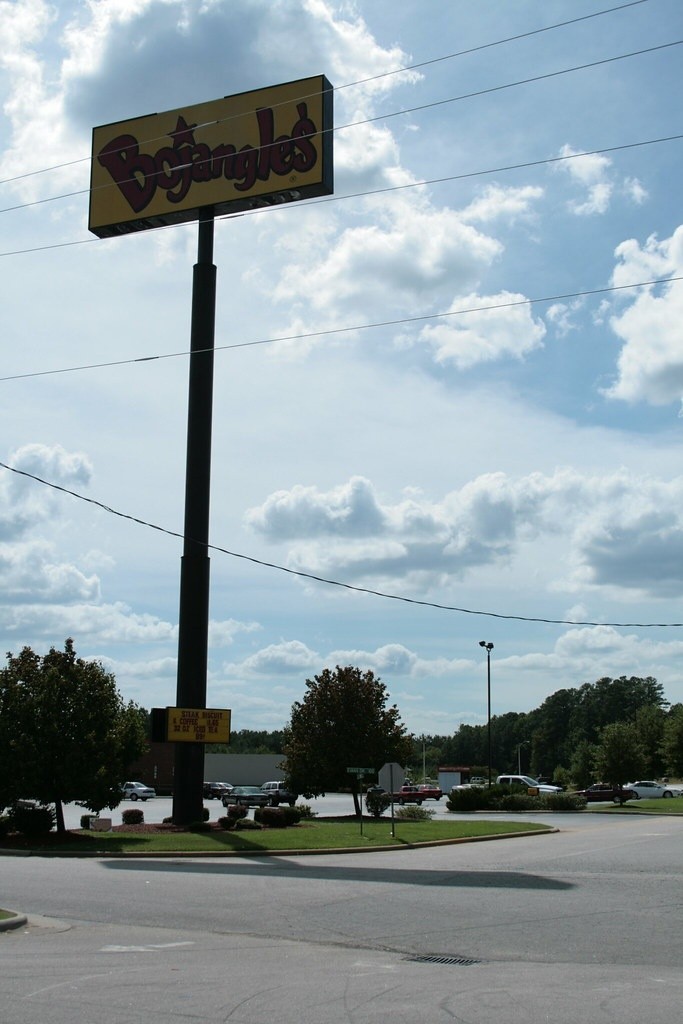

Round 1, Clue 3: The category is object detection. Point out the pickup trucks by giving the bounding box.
[451,774,564,795]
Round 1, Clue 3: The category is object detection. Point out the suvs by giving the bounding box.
[260,781,299,807]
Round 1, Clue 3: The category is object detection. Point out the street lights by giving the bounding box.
[518,741,530,776]
[479,640,495,792]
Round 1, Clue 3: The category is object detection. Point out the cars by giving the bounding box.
[622,780,681,799]
[380,785,427,806]
[570,783,633,803]
[222,785,271,809]
[203,781,235,801]
[120,781,157,801]
[417,784,443,801]
